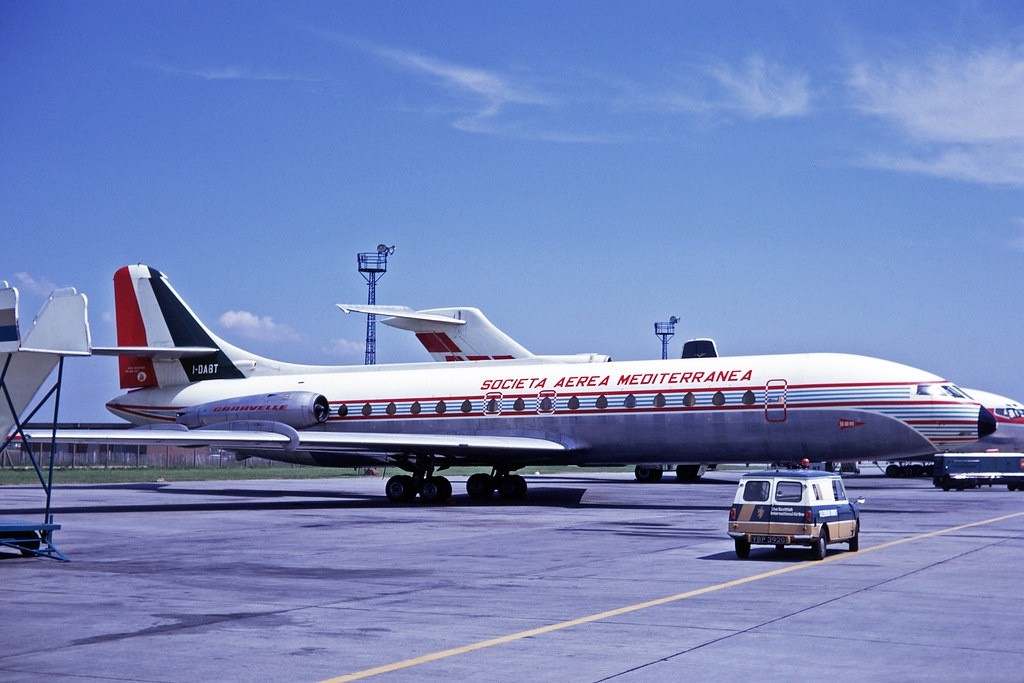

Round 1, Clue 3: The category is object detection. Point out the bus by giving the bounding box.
[932,448,1024,492]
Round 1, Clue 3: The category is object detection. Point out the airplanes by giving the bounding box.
[80,265,998,506]
[878,386,1024,478]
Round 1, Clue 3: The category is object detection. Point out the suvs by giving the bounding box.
[727,459,866,561]
